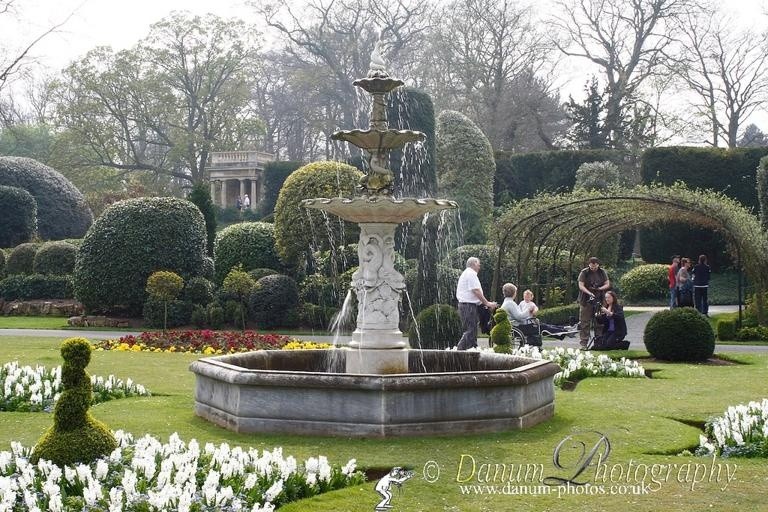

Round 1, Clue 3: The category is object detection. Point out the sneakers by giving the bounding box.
[567,324,578,338]
[579,345,588,351]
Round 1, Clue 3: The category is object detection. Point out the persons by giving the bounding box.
[501,284,578,341]
[595,290,631,351]
[455,256,497,349]
[519,289,539,318]
[244,194,250,207]
[576,256,610,350]
[236,196,242,210]
[669,253,710,314]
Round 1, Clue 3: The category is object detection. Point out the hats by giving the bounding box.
[589,257,600,263]
[672,255,680,259]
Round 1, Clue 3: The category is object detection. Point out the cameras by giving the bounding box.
[594,301,602,313]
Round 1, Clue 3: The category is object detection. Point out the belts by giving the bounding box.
[463,303,475,304]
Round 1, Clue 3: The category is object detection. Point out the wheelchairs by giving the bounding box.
[482,303,543,355]
